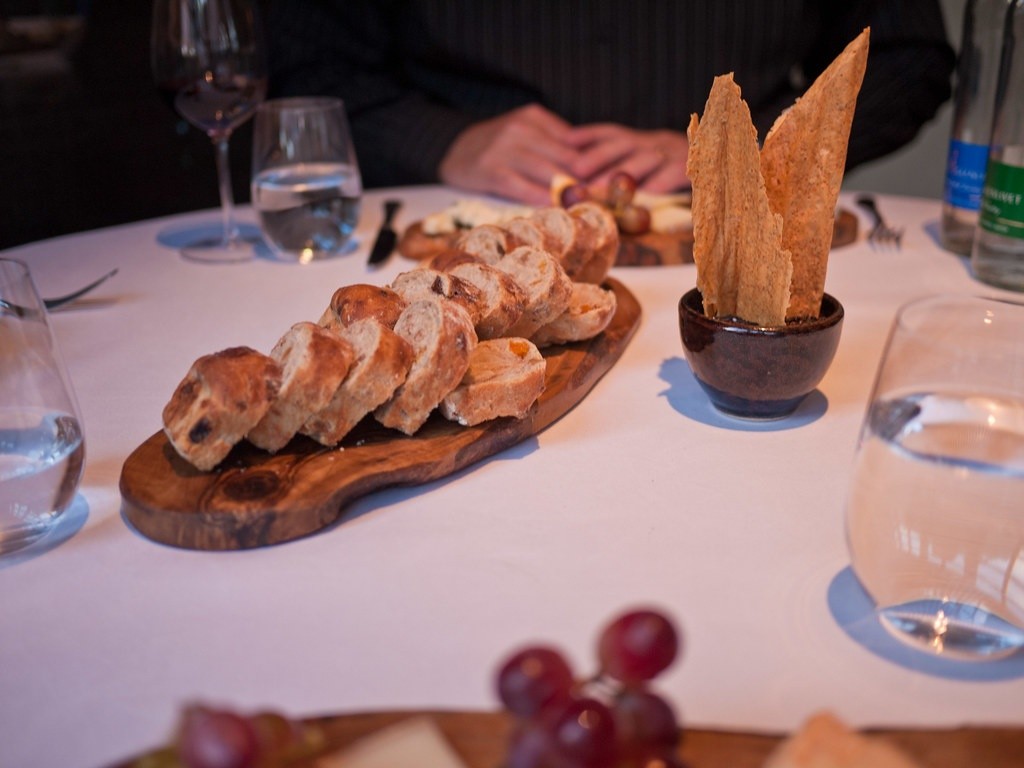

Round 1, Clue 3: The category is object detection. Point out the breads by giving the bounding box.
[753,711,917,768]
[162,295,549,472]
[549,169,694,236]
[416,201,620,287]
[686,72,793,327]
[761,27,870,321]
[319,246,618,350]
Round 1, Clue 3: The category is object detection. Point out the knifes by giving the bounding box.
[366,199,402,267]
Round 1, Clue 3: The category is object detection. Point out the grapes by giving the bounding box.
[559,171,651,236]
[180,704,321,768]
[496,608,691,768]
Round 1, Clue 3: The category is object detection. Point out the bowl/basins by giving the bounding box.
[678,285,844,420]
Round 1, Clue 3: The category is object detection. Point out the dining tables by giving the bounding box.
[1,164,1023,767]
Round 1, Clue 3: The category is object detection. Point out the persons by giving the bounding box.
[256,1,955,211]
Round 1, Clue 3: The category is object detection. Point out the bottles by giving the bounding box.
[941,0,1018,256]
[971,1,1024,290]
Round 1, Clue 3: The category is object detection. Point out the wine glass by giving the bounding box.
[149,1,273,263]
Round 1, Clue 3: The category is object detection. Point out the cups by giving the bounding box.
[1,257,84,560]
[843,291,1024,660]
[250,98,363,262]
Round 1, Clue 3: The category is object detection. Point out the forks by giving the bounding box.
[851,194,907,245]
[0,270,122,318]
